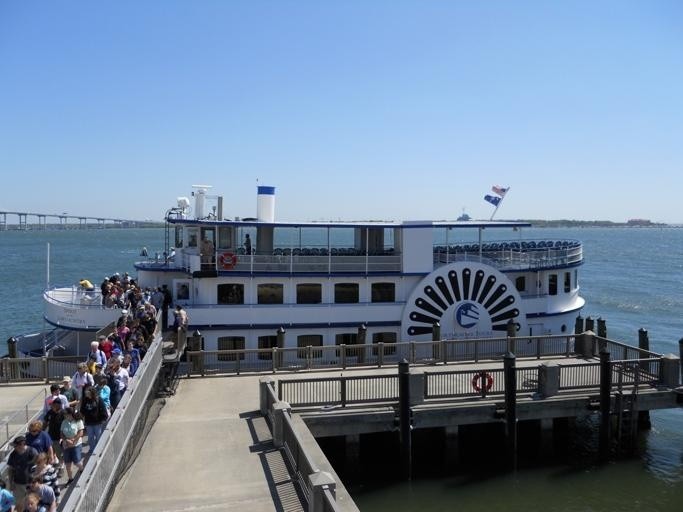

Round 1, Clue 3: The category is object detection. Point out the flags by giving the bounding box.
[483,195,503,206]
[491,183,508,197]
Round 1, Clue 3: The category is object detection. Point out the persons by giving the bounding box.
[79,273,188,406]
[243,233,252,253]
[0,362,111,512]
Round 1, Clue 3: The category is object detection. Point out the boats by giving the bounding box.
[4,185,587,380]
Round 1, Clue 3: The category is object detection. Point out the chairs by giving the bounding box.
[238,245,366,257]
[433,239,579,267]
